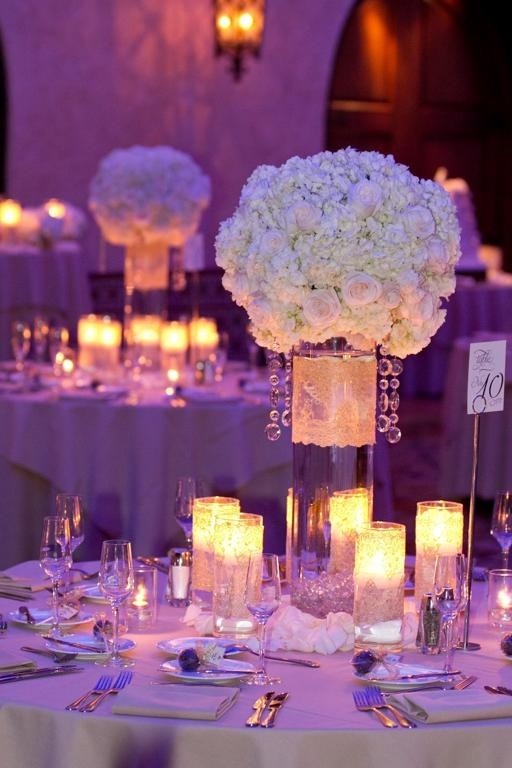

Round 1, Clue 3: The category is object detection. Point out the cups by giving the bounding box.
[354,521,407,655]
[489,568,512,637]
[328,485,369,578]
[213,512,264,647]
[74,313,220,382]
[123,566,156,629]
[415,501,464,627]
[193,497,241,616]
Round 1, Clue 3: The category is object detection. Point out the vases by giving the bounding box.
[289,354,377,613]
[122,247,172,317]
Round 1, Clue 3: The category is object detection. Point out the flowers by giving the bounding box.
[87,143,218,247]
[216,147,464,358]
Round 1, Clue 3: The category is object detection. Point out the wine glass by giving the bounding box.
[99,539,135,668]
[491,490,512,570]
[55,495,84,558]
[12,321,32,392]
[40,516,74,638]
[432,553,468,673]
[242,554,282,687]
[174,477,197,551]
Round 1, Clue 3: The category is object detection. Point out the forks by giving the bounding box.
[366,685,414,728]
[386,676,478,693]
[81,670,133,713]
[65,674,113,711]
[20,645,78,663]
[68,564,112,581]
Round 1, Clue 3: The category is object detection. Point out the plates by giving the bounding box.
[154,636,235,657]
[352,671,440,690]
[45,634,135,660]
[162,659,254,686]
[10,610,94,630]
[60,387,129,401]
[187,392,246,404]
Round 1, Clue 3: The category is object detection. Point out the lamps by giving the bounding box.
[211,2,265,82]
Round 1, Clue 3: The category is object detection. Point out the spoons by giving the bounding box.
[225,642,320,669]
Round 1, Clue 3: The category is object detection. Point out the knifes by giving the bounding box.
[351,688,395,727]
[261,692,290,728]
[137,556,169,575]
[0,665,83,684]
[245,691,275,728]
[45,638,103,652]
[158,668,263,676]
